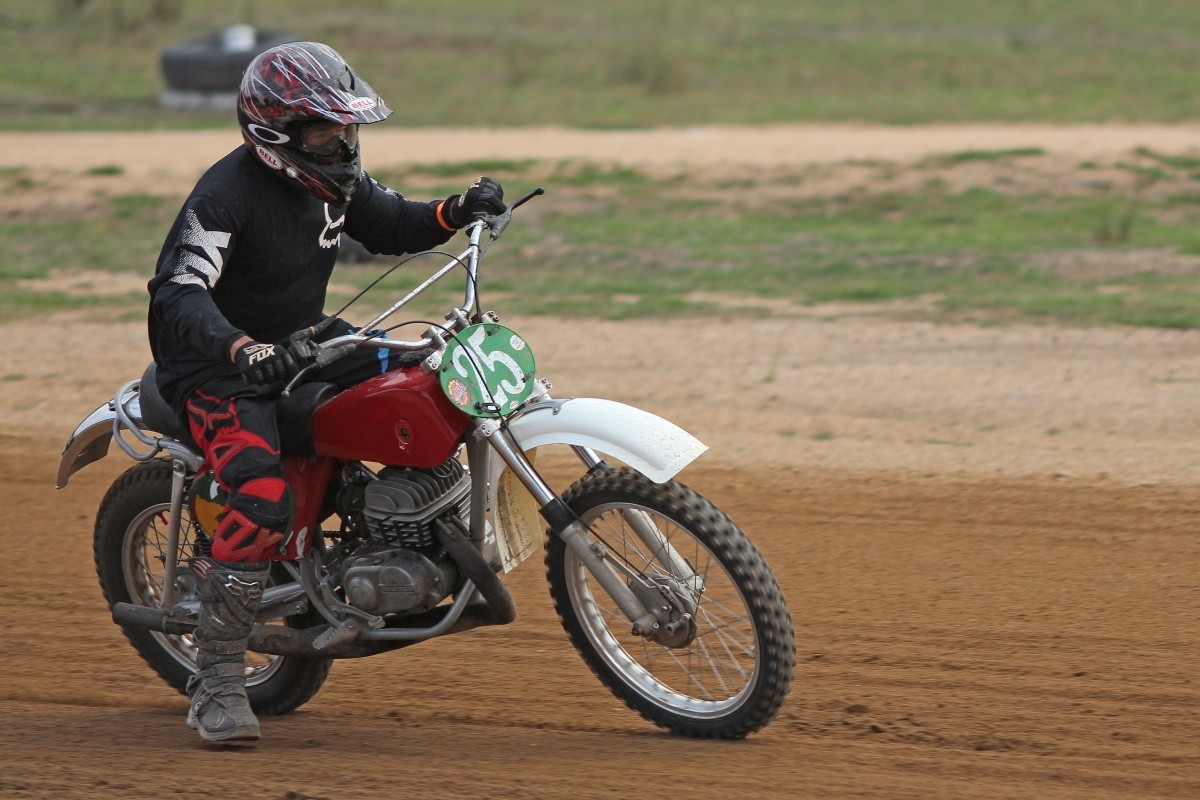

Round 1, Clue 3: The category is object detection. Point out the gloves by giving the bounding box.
[441,176,506,229]
[232,342,301,387]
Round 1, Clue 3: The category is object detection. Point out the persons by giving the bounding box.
[146,40,507,737]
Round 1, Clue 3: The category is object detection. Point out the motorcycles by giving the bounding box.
[54,188,798,741]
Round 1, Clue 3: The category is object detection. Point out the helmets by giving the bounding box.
[236,43,394,206]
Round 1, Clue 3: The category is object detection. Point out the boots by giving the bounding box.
[186,555,272,745]
